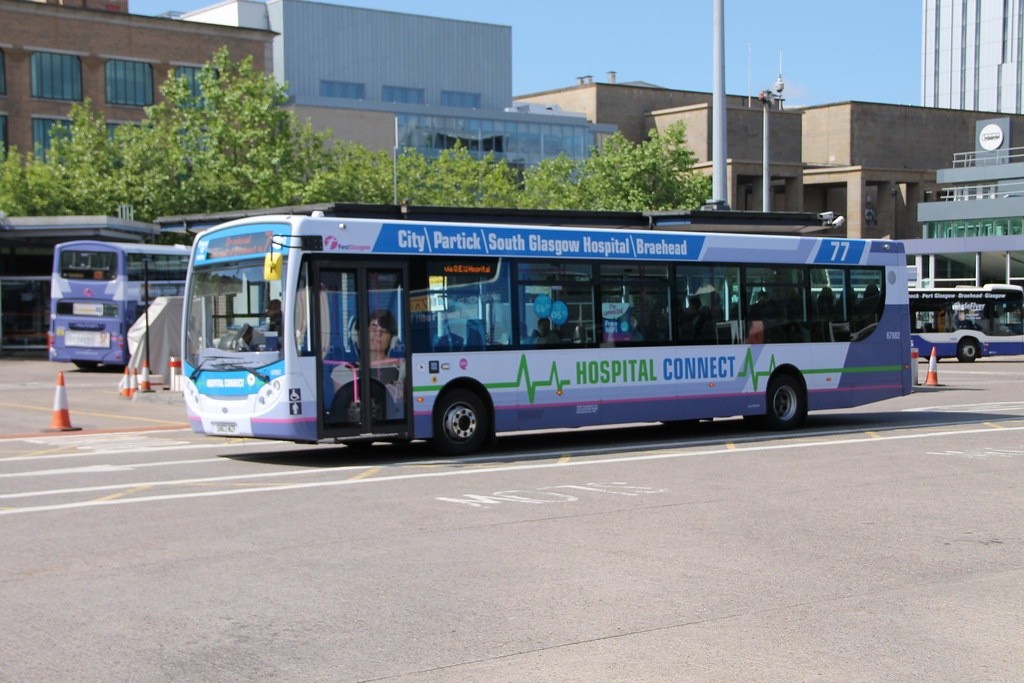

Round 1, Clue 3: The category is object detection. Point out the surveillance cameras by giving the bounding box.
[817,211,833,219]
[833,216,844,229]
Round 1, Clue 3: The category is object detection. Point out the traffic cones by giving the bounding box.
[139,359,156,393]
[122,367,130,398]
[918,346,946,387]
[39,372,82,432]
[129,368,139,397]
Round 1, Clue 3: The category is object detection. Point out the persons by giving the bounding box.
[750,282,879,343]
[955,310,974,330]
[643,290,731,344]
[332,310,401,402]
[533,317,561,345]
[264,300,283,347]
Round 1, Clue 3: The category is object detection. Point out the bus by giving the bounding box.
[181,210,912,456]
[908,284,1024,362]
[48,240,194,371]
